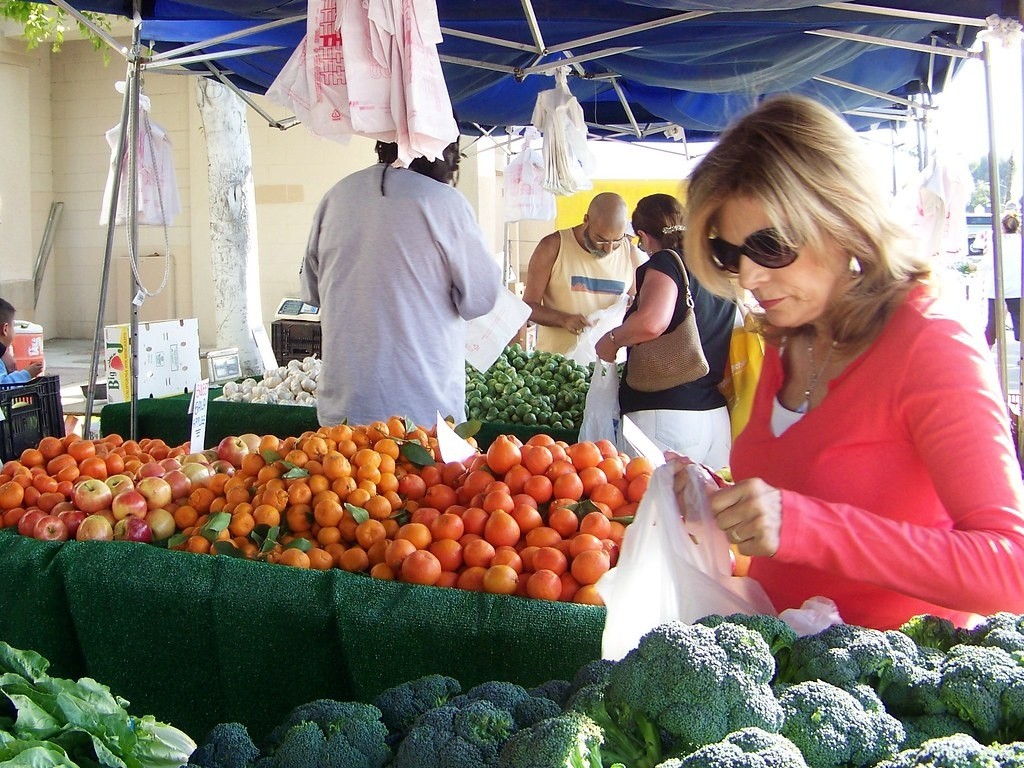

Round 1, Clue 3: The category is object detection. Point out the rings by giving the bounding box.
[731,527,745,542]
[578,329,582,333]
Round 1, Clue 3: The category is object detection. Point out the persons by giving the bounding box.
[681,95,1024,631]
[523,191,639,367]
[298,112,502,432]
[0,298,44,391]
[594,193,745,472]
[983,213,1022,350]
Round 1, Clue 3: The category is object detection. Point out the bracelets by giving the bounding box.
[609,331,620,346]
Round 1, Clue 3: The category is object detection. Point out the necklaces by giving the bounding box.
[796,332,838,414]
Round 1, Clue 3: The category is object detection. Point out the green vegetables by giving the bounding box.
[0,640,198,768]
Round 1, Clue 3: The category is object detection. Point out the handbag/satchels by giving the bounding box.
[626,248,711,392]
[578,353,627,455]
[598,458,776,661]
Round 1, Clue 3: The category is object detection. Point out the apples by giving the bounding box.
[19,434,262,545]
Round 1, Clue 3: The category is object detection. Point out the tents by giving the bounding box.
[24,0,1024,457]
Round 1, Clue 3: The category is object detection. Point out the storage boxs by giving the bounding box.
[271,319,322,369]
[103,317,202,405]
[0,375,66,464]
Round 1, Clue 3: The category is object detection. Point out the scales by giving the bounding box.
[274,297,323,322]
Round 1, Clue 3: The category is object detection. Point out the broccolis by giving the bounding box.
[178,610,1024,768]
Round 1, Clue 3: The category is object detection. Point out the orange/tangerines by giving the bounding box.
[0,434,194,529]
[168,415,753,606]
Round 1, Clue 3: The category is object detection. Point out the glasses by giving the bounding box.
[709,230,807,274]
[586,223,626,246]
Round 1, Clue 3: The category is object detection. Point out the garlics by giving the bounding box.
[223,353,323,406]
